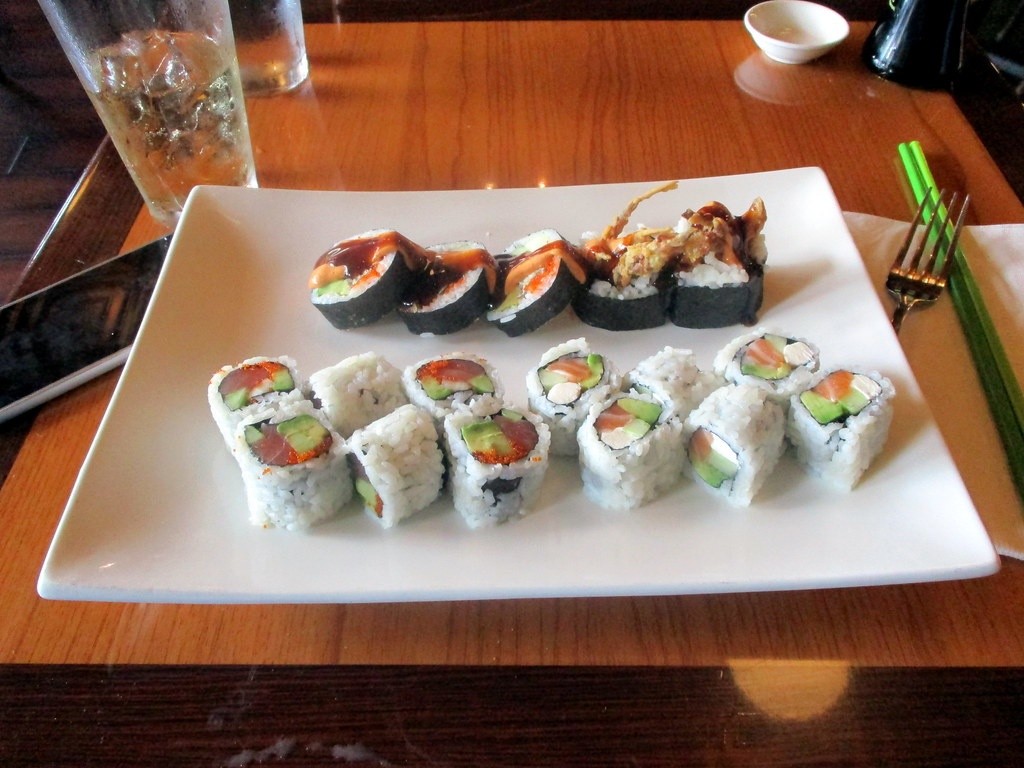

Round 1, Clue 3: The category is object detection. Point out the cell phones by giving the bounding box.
[0,231,174,422]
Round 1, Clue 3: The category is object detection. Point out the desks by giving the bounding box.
[0,22,1024,768]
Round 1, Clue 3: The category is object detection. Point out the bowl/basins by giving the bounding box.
[743,0,851,65]
[733,50,815,106]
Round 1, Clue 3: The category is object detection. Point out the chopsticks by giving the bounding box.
[897,140,1024,511]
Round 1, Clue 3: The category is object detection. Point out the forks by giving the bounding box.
[883,182,973,337]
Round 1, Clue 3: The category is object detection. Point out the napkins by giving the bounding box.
[844,210,1024,565]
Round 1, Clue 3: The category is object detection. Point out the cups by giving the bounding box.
[857,0,973,91]
[228,0,309,98]
[34,0,260,223]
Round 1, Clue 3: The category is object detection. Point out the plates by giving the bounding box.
[35,163,1003,608]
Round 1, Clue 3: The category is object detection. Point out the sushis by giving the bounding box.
[308,179,770,338]
[208,329,897,530]
